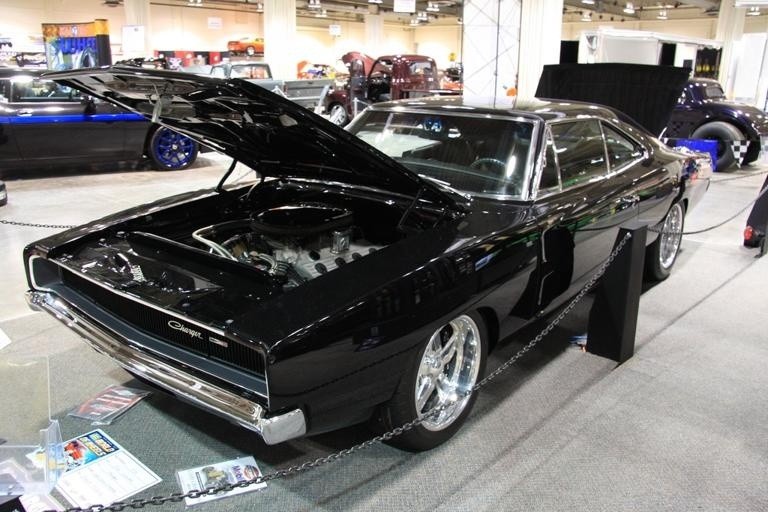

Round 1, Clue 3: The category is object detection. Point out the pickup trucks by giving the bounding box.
[193,63,337,115]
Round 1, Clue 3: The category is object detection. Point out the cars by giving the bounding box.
[227,34,265,56]
[664,75,768,172]
[21,63,714,450]
[326,51,464,130]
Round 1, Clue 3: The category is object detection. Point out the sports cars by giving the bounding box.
[0,65,216,175]
